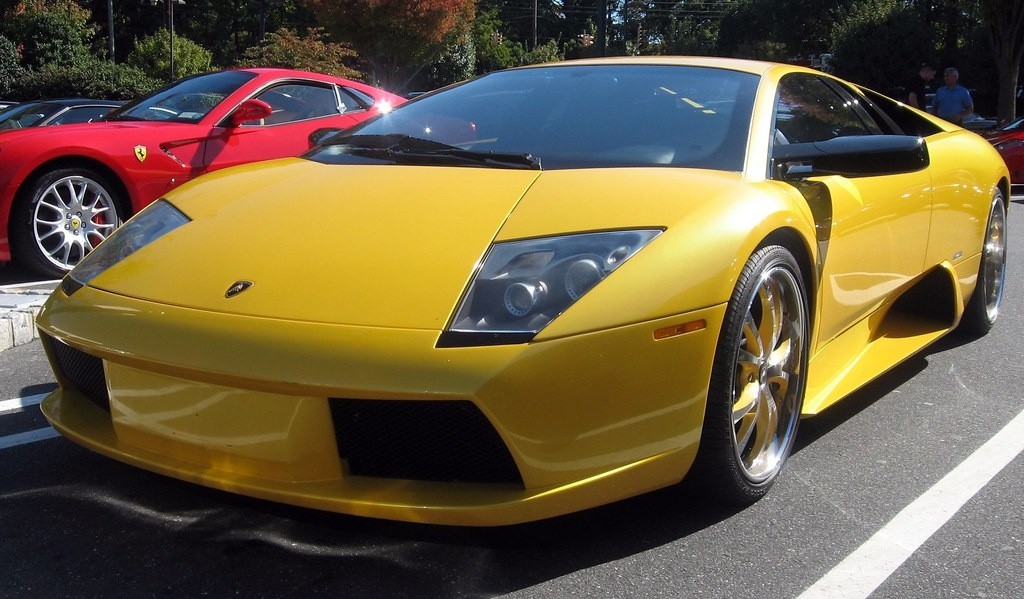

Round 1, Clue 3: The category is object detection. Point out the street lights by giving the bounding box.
[150,0,186,83]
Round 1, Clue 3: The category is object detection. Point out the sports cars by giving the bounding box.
[35,56,1010,530]
[971,116,1024,184]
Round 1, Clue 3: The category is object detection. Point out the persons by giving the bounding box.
[932,67,975,124]
[905,64,937,112]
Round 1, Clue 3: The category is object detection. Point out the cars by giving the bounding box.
[0,67,478,282]
[0,104,59,130]
[0,98,181,131]
